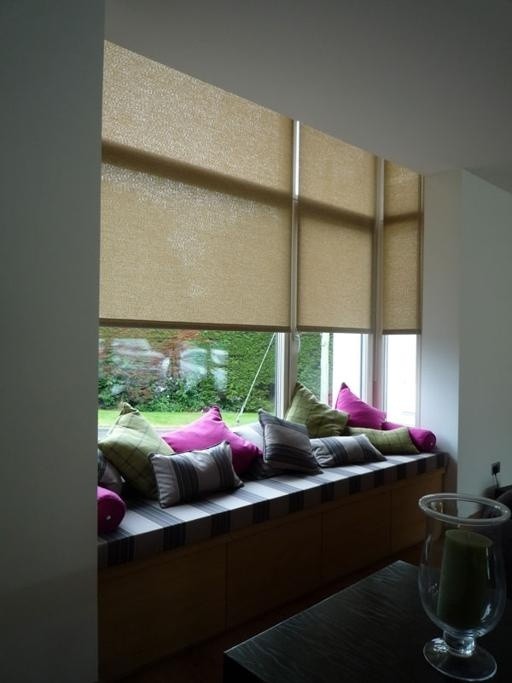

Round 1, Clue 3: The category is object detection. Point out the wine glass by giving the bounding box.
[419,493,512,682]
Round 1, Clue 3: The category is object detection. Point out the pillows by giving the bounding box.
[343,424,418,454]
[228,421,264,453]
[97,486,127,536]
[98,401,175,500]
[335,382,386,429]
[146,441,243,508]
[160,405,264,472]
[382,421,436,451]
[258,408,323,474]
[283,381,349,438]
[97,449,132,497]
[308,433,388,468]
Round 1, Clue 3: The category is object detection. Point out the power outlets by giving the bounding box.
[491,460,501,475]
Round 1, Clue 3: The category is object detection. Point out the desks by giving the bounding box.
[223,559,511,682]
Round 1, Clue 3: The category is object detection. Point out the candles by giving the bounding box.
[437,527,493,629]
